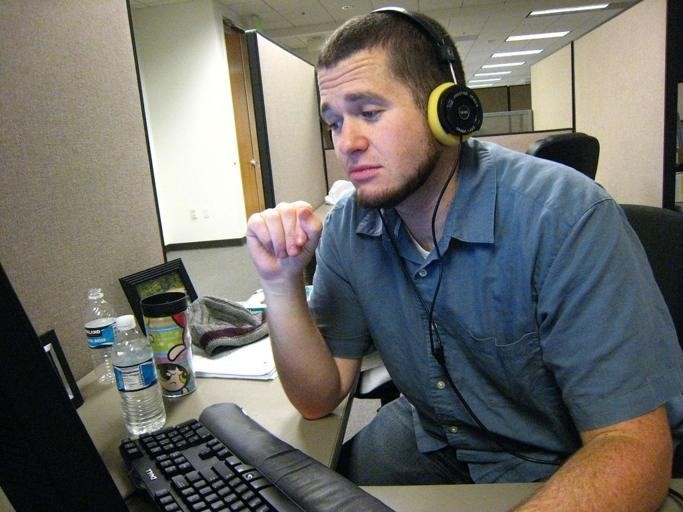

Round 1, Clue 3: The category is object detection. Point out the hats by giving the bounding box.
[186,294,270,357]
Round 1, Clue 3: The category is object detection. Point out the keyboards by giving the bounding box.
[118,418,304,512]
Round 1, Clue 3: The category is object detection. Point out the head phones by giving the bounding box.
[370,3,483,147]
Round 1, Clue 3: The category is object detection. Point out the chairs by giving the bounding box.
[525,132,600,180]
[354,201,682,478]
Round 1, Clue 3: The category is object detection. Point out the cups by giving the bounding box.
[141,294,204,400]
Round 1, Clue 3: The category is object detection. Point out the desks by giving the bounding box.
[1,301,682,512]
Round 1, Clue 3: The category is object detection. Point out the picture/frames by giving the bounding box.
[118,258,199,337]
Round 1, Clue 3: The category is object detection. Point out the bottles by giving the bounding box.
[82,282,120,387]
[108,316,167,436]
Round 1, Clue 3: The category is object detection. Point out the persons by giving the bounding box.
[247,10,681,511]
